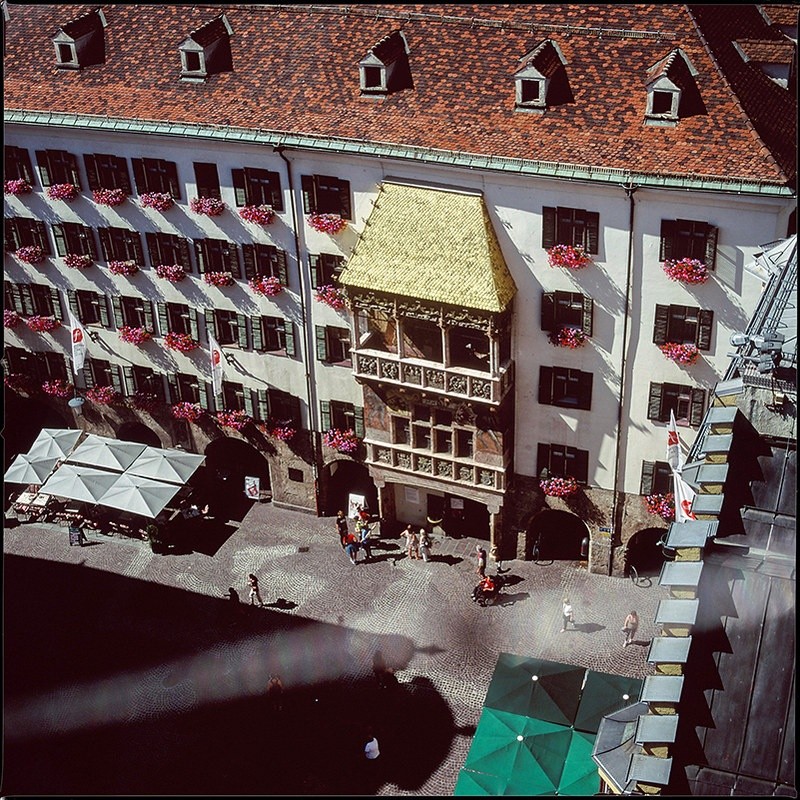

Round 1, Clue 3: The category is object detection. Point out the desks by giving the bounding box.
[65,500,83,513]
[16,492,37,505]
[33,494,51,507]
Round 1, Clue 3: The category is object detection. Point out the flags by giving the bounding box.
[667,412,681,473]
[68,312,86,375]
[674,474,696,522]
[208,337,222,395]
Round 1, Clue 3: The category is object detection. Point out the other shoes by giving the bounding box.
[629,639,632,644]
[560,629,565,632]
[572,623,576,628]
[623,642,628,648]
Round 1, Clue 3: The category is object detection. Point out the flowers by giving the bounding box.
[245,272,283,298]
[536,473,580,501]
[544,321,589,351]
[4,177,35,198]
[189,195,228,218]
[106,258,142,279]
[155,264,188,284]
[661,254,712,291]
[3,309,354,460]
[45,181,83,204]
[62,253,93,270]
[305,211,348,236]
[90,186,127,205]
[315,285,345,310]
[656,338,701,369]
[138,192,174,213]
[203,270,235,289]
[239,206,277,227]
[645,492,677,523]
[15,243,43,264]
[546,240,591,274]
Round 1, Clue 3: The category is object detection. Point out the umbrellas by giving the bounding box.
[4,428,207,519]
[453,653,642,796]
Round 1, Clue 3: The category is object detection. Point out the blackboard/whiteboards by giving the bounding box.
[68,527,85,543]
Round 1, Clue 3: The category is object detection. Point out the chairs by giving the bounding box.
[6,484,133,539]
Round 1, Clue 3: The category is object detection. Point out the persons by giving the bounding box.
[70,517,87,541]
[622,610,638,648]
[560,598,575,633]
[475,545,486,580]
[336,511,348,549]
[528,533,544,564]
[353,507,373,559]
[400,525,431,562]
[470,575,495,602]
[342,530,358,564]
[268,673,283,711]
[373,651,386,686]
[363,734,380,761]
[248,574,263,607]
[229,588,239,602]
